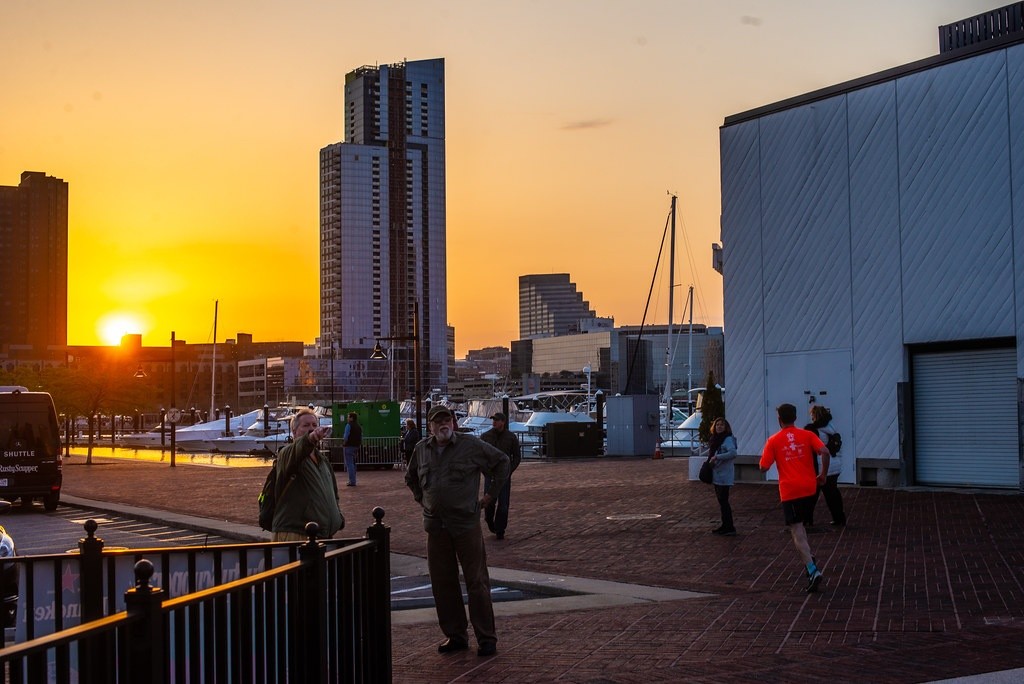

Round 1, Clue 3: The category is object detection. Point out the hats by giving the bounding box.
[489,413,506,420]
[428,406,451,420]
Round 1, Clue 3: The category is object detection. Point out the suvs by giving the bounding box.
[0,384,66,512]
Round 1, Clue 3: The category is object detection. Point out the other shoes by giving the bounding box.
[488,522,496,533]
[829,521,845,528]
[495,530,504,541]
[347,482,356,486]
[805,553,817,577]
[711,527,738,536]
[805,574,824,594]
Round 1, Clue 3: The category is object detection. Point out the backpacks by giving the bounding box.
[258,446,284,531]
[821,425,842,457]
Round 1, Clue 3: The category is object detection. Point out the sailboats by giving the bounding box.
[74,195,724,465]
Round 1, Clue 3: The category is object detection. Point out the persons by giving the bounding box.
[805,405,847,530]
[342,413,361,486]
[759,404,831,594]
[405,405,511,657]
[479,413,521,539]
[401,420,420,465]
[707,418,737,536]
[270,410,344,541]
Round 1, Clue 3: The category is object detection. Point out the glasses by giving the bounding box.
[430,416,453,422]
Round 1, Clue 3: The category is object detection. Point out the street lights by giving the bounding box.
[370,302,422,445]
[133,331,177,468]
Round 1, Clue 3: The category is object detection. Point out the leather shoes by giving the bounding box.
[477,646,496,655]
[438,639,469,650]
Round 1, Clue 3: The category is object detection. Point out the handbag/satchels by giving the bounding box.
[699,461,714,485]
